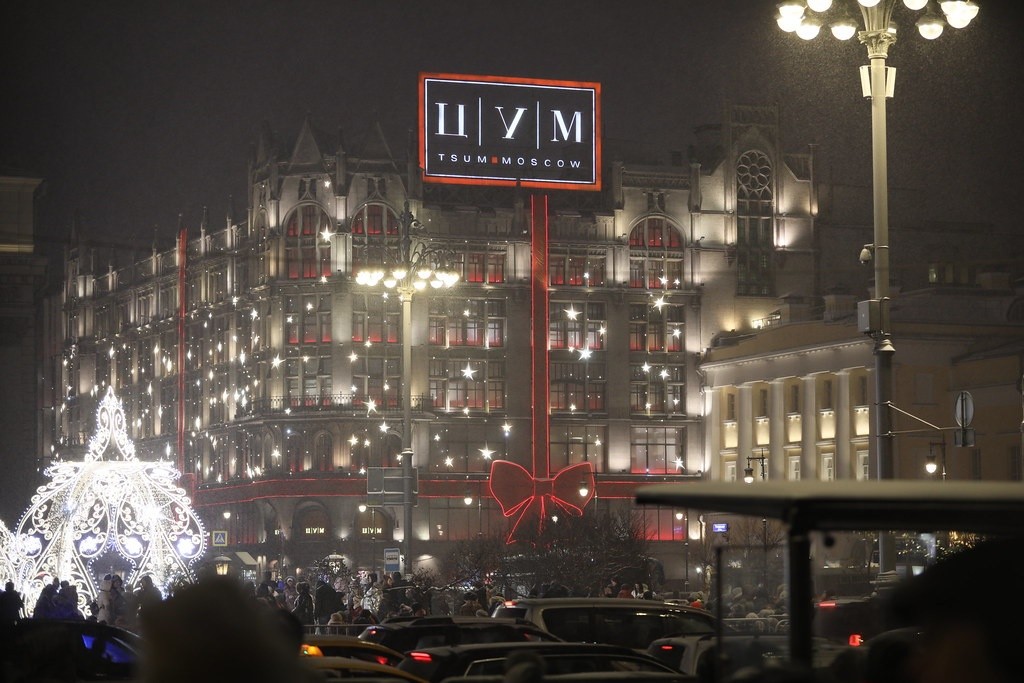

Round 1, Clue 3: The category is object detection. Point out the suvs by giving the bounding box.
[871,537,925,563]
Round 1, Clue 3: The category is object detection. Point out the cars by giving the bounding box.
[0,552,911,681]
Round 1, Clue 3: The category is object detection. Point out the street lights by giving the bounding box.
[744,448,769,588]
[925,432,949,549]
[358,495,376,575]
[775,0,981,580]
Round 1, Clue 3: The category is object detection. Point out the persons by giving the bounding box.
[0,526,1024,683]
